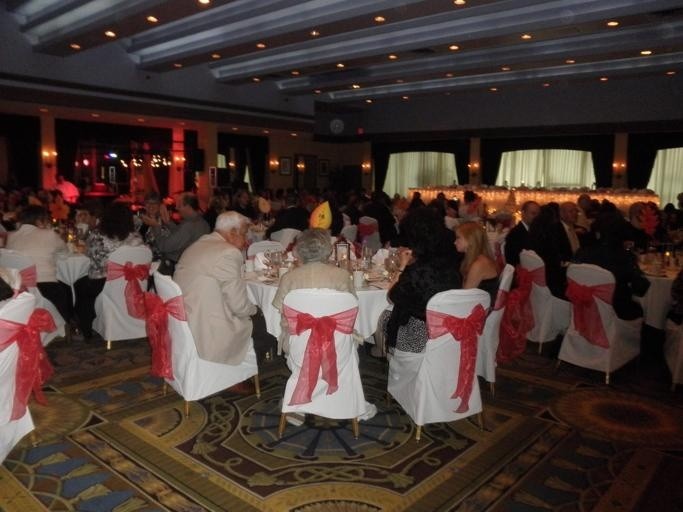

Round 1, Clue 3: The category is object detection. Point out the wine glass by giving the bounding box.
[41,211,107,278]
[631,241,683,277]
[254,243,403,292]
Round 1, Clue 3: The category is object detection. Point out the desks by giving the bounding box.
[1,241,170,320]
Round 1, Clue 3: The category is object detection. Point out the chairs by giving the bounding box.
[244,212,682,389]
[387,288,490,444]
[0,248,66,468]
[278,288,366,440]
[93,245,153,350]
[132,268,260,419]
[477,264,531,399]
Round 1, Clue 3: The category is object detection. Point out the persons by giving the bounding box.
[270,228,359,356]
[0,182,680,357]
[168,209,288,367]
